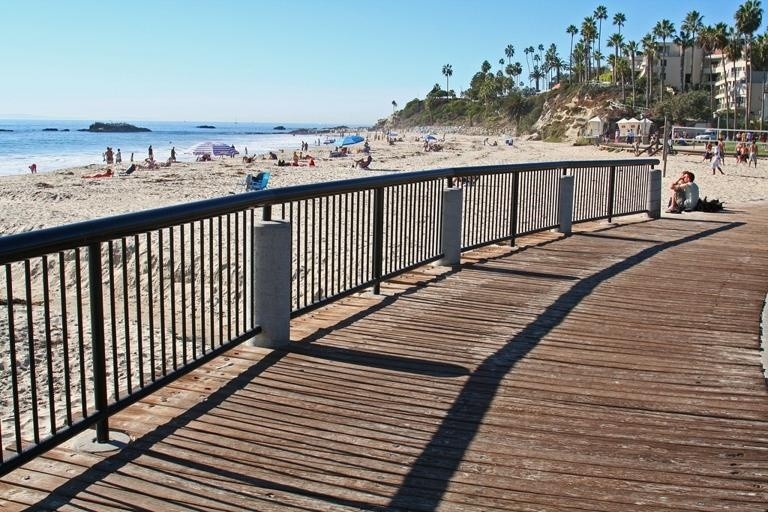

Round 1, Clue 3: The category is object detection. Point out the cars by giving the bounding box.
[696,130,716,142]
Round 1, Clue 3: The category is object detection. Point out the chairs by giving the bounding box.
[244,172,270,191]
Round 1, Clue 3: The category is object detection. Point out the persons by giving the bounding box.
[317,124,525,154]
[609,124,768,168]
[710,140,726,176]
[679,171,689,185]
[28,164,36,174]
[95,139,377,178]
[666,172,699,214]
